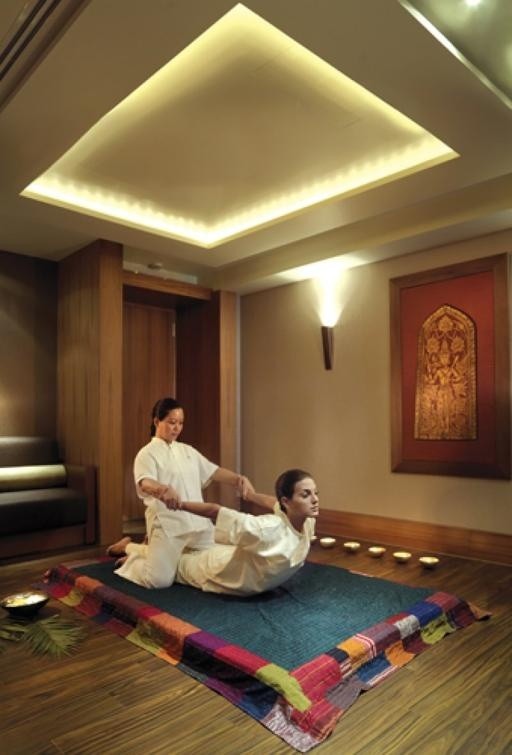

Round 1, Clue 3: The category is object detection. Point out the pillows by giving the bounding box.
[1,463,66,490]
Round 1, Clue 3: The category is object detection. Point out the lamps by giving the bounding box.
[320,327,335,371]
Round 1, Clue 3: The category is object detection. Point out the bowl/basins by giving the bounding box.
[392,551,411,563]
[307,535,317,544]
[343,541,361,553]
[418,555,439,568]
[1,591,51,619]
[319,537,337,548]
[367,546,386,558]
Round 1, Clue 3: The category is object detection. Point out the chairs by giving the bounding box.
[1,433,98,560]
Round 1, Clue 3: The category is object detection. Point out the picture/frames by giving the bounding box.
[387,253,512,484]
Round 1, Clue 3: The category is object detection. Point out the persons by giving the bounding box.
[104,468,320,601]
[114,397,254,596]
